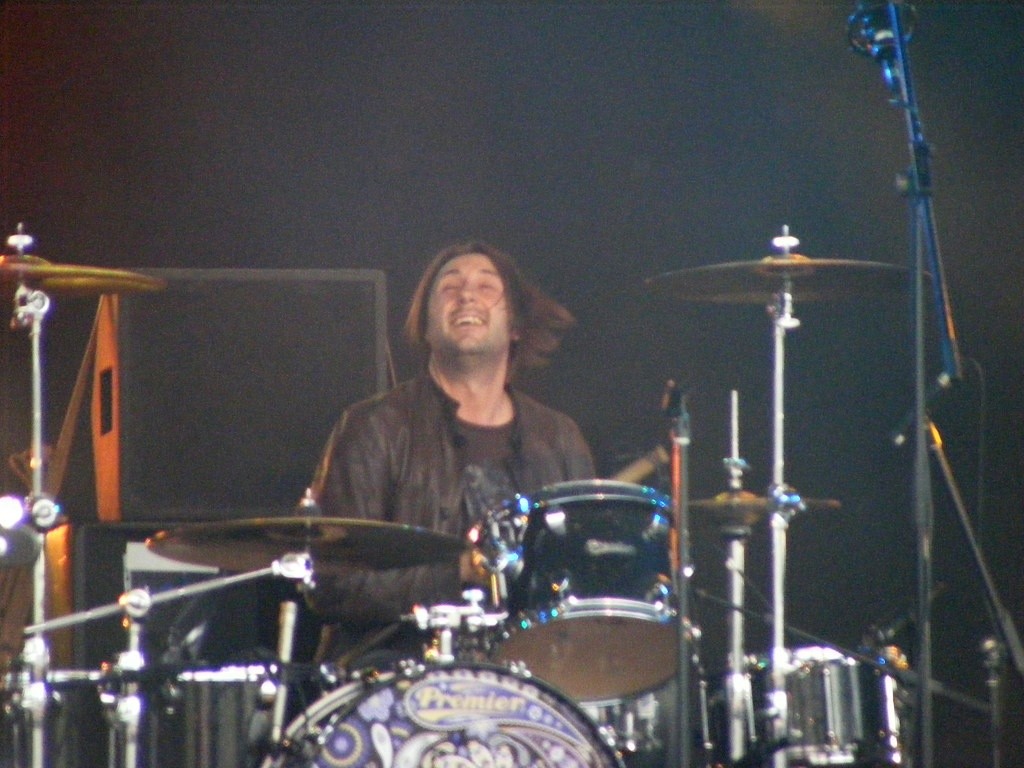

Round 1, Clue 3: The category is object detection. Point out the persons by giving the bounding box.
[306,238,602,706]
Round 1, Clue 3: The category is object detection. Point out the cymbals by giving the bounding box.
[684,255,937,306]
[146,515,466,573]
[688,488,843,528]
[0,253,168,296]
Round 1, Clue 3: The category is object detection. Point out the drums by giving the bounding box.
[713,646,889,766]
[471,477,681,704]
[152,661,264,768]
[260,660,627,768]
[0,670,125,768]
